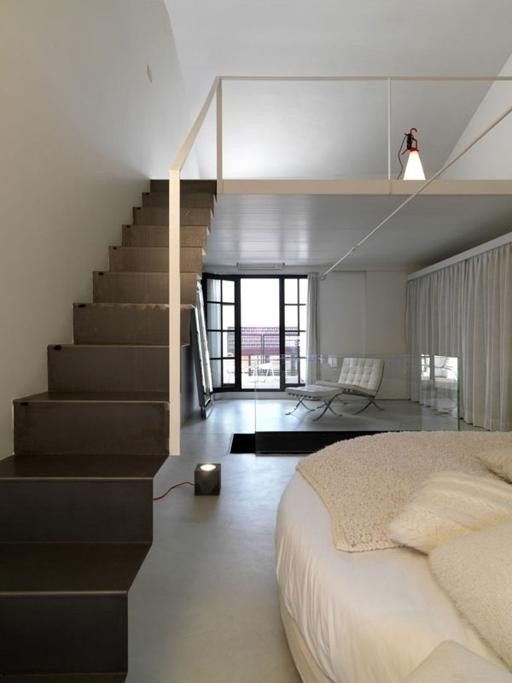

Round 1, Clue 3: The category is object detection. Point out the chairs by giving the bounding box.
[283,355,387,421]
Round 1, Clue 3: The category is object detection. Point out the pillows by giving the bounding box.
[386,465,512,556]
[477,442,512,481]
[422,517,511,663]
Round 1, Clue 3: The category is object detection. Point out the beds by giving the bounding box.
[271,425,511,683]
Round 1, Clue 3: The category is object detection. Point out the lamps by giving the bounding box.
[395,123,428,181]
[153,462,222,500]
